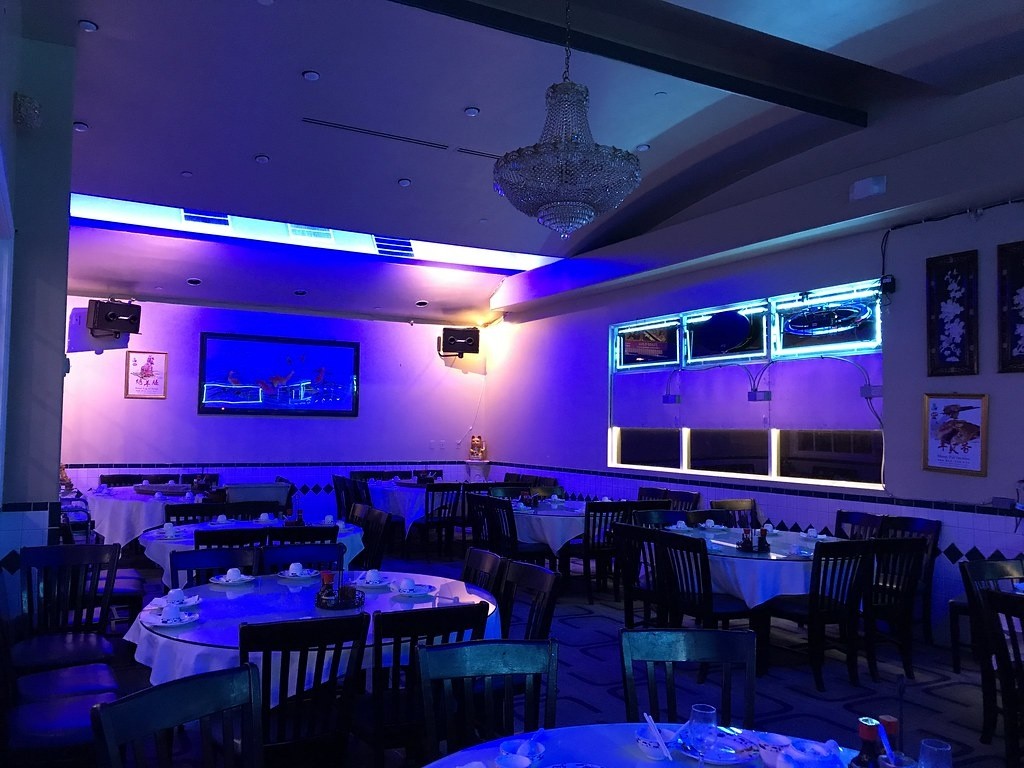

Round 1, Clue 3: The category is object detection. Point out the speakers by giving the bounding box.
[442,327,479,354]
[86,300,141,333]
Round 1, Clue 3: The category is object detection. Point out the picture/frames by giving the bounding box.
[923,391,990,479]
[925,248,978,376]
[996,240,1024,373]
[125,350,169,400]
[197,331,359,417]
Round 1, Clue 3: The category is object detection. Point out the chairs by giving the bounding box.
[758,509,944,691]
[958,558,1024,766]
[0,470,757,768]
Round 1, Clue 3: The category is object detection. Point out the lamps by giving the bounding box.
[494,12,642,241]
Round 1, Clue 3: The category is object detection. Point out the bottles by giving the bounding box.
[878,714,899,755]
[316,571,338,610]
[775,740,845,768]
[848,716,884,768]
[741,528,767,552]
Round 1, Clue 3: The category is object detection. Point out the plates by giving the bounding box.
[357,577,396,588]
[799,531,826,540]
[159,596,202,607]
[277,568,320,580]
[672,727,760,766]
[209,574,255,585]
[398,584,437,597]
[664,525,693,533]
[699,523,728,531]
[752,529,779,536]
[149,613,199,628]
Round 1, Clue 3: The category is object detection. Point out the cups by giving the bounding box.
[918,738,953,768]
[260,513,268,521]
[764,524,773,533]
[226,568,241,582]
[218,515,226,523]
[143,480,149,485]
[160,604,182,624]
[878,754,918,768]
[551,495,557,502]
[757,732,791,768]
[706,520,714,528]
[634,727,676,761]
[364,570,381,584]
[154,492,162,500]
[289,563,302,577]
[808,529,817,538]
[164,526,175,539]
[169,480,174,484]
[400,578,416,594]
[186,492,193,499]
[325,515,333,525]
[164,523,173,531]
[676,520,685,529]
[167,588,184,605]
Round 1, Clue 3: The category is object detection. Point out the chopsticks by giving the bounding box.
[644,712,672,761]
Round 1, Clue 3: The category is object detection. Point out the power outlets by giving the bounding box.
[456,439,461,448]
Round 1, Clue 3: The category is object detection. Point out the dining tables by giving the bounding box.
[494,497,626,595]
[123,570,501,704]
[655,526,870,673]
[88,487,223,557]
[139,519,364,588]
[421,722,882,768]
[367,479,484,543]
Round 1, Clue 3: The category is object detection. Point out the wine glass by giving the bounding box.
[689,704,717,768]
